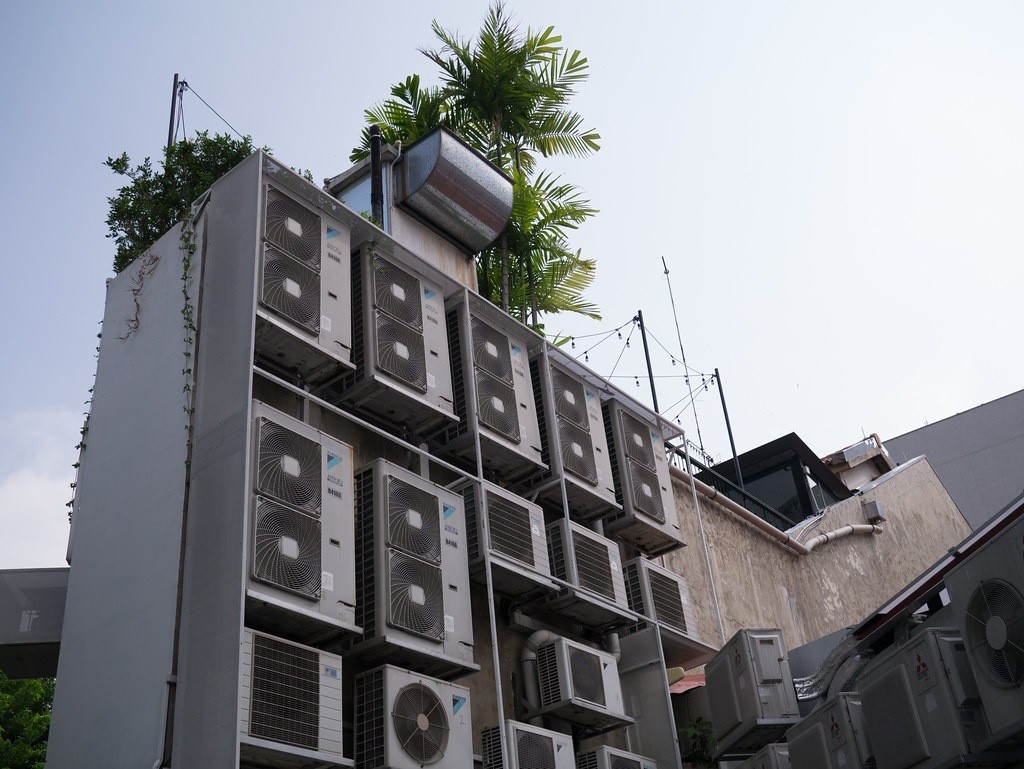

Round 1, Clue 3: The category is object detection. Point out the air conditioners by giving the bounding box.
[255,171,351,385]
[704,629,800,750]
[535,636,625,729]
[244,398,356,644]
[856,626,988,769]
[543,519,628,629]
[339,240,454,436]
[739,743,792,769]
[622,556,699,640]
[338,458,473,678]
[239,627,343,769]
[354,664,474,769]
[449,481,551,597]
[436,303,542,480]
[786,692,878,769]
[602,398,680,551]
[577,744,657,769]
[943,514,1024,735]
[480,719,576,769]
[522,353,616,520]
